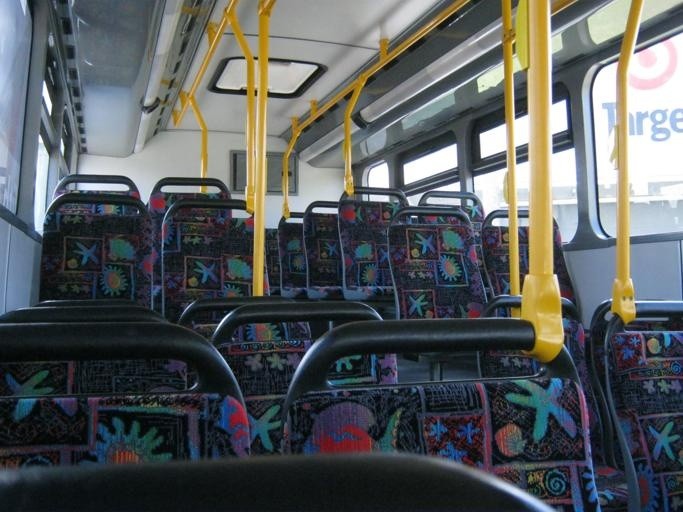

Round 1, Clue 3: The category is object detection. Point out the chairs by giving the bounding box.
[2,174,682,509]
